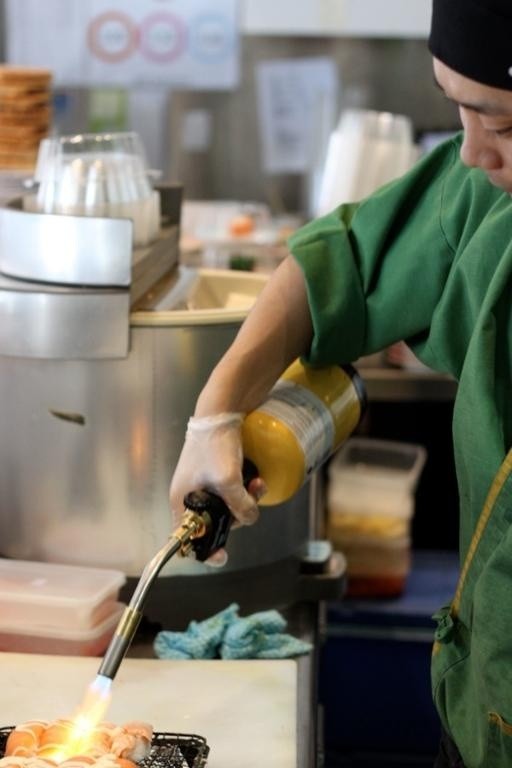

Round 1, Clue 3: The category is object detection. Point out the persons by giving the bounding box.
[167,2,511,766]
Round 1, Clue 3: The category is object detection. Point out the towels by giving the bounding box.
[147,602,316,664]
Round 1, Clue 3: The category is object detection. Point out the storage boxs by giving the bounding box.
[319,547,463,764]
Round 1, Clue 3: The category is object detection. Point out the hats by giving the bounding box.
[426,0,512,93]
